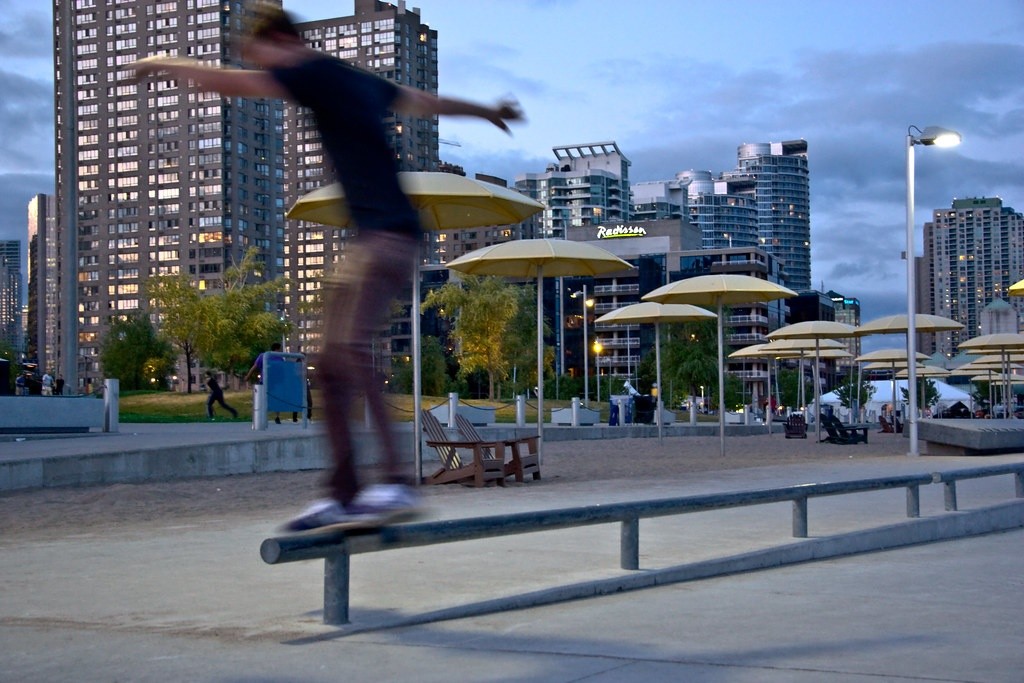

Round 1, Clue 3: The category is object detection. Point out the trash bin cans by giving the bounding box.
[263,350,306,414]
[635,395,656,424]
[609,395,633,428]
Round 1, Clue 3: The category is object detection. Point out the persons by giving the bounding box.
[14,370,65,396]
[123,2,532,540]
[244,343,282,424]
[203,370,240,419]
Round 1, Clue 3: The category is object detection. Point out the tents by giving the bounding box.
[813,380,975,423]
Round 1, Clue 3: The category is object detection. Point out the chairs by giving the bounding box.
[827,413,870,445]
[453,412,543,482]
[814,412,858,443]
[420,409,508,488]
[781,413,809,438]
[889,415,904,434]
[877,415,894,434]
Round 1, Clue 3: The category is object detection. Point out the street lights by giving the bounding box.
[594,343,601,402]
[570,284,595,406]
[699,385,704,414]
[906,122,963,455]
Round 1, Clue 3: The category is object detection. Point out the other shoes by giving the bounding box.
[286,499,348,532]
[340,485,414,521]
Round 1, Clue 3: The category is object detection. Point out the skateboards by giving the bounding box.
[285,498,421,526]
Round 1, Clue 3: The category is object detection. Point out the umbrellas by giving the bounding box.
[444,239,640,464]
[640,274,799,458]
[285,172,547,483]
[595,302,718,448]
[727,280,1024,444]
